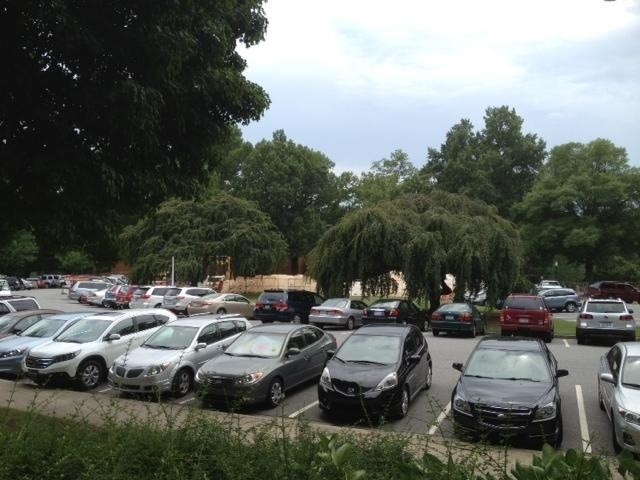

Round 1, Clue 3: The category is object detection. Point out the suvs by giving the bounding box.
[251,285,326,325]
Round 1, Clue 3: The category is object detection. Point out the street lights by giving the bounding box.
[215,255,231,271]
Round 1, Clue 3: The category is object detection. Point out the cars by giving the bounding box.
[307,295,369,331]
[594,340,640,461]
[360,297,430,333]
[191,320,338,412]
[428,301,488,340]
[315,321,434,420]
[0,270,258,401]
[447,333,570,451]
[463,277,640,343]
